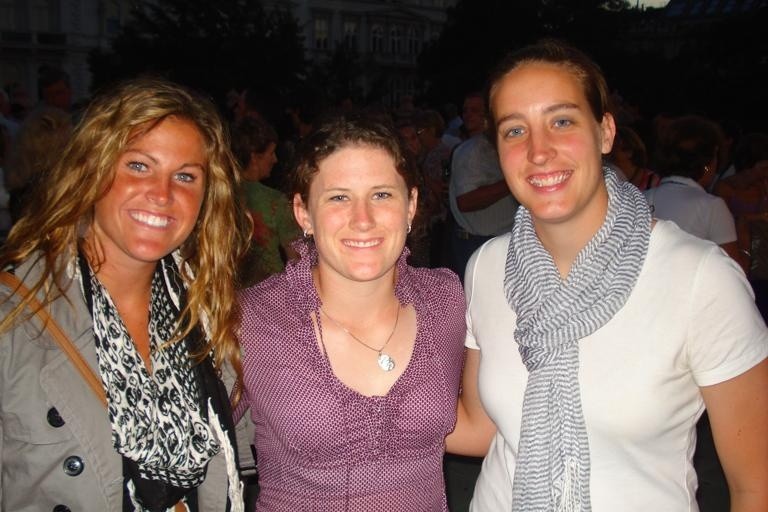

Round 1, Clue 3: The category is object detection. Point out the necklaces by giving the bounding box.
[320,305,402,373]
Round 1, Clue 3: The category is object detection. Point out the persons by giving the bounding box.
[1,66,767,323]
[440,37,768,512]
[0,74,262,512]
[233,115,467,512]
